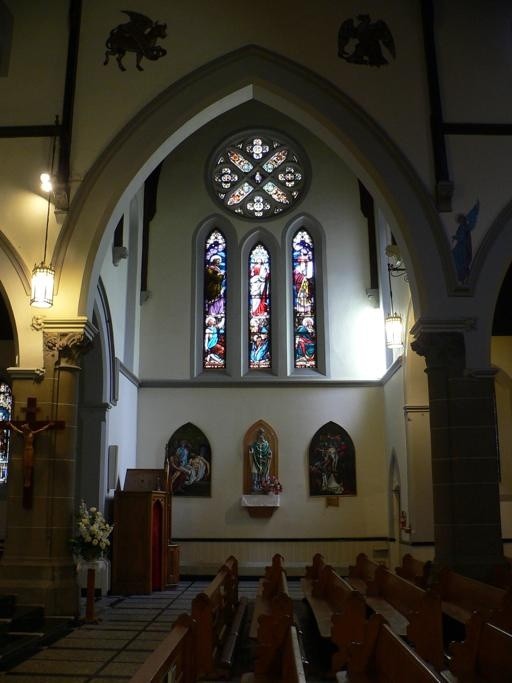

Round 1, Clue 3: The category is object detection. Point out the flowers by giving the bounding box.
[70,501,118,560]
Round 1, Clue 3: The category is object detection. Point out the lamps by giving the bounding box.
[384,264,407,348]
[28,115,60,308]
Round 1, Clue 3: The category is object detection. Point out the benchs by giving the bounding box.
[131,553,511,683]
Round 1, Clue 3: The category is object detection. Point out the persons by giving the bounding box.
[6,417,54,489]
[452,210,476,284]
[249,433,273,490]
[170,437,210,485]
[205,257,314,368]
